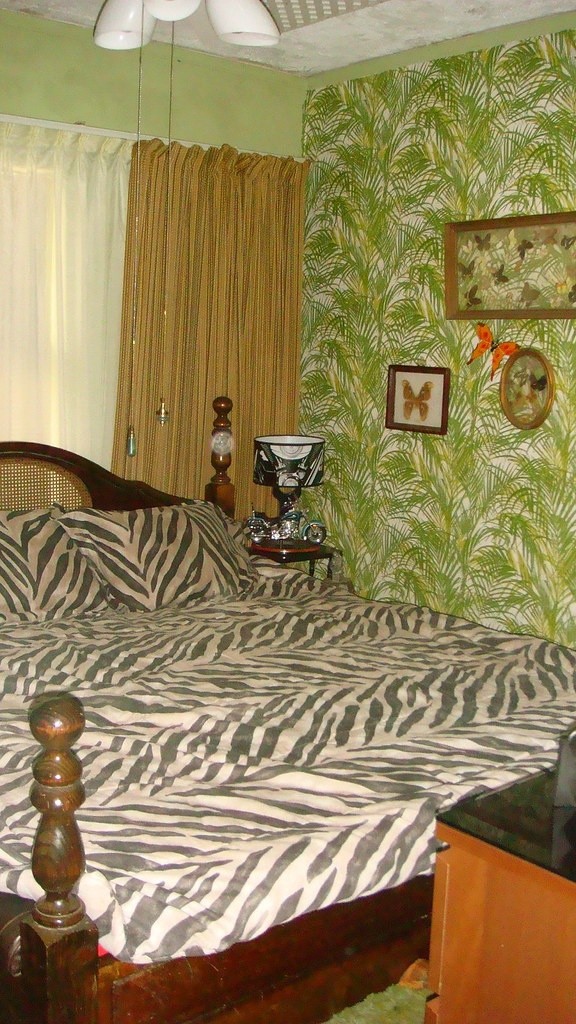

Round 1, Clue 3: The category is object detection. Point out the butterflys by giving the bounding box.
[507,360,547,414]
[457,228,576,307]
[402,379,432,421]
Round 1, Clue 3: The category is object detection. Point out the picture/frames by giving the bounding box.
[444,210,576,321]
[386,365,451,435]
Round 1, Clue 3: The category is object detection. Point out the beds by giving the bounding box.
[0,441,576,1024]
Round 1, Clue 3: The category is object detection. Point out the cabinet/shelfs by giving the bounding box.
[422,765,576,1024]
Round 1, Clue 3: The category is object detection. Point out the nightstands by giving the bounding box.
[251,538,342,580]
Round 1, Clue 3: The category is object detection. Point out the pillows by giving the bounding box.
[0,502,119,626]
[57,499,261,615]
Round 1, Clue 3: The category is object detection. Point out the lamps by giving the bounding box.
[94,0,281,52]
[254,434,324,527]
